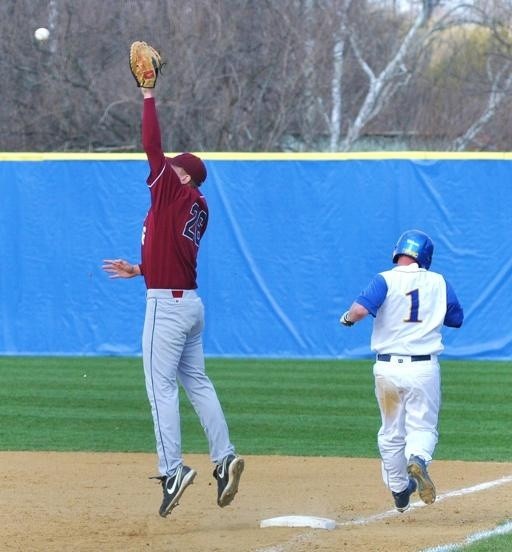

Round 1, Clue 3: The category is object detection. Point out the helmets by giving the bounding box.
[392,230,434,271]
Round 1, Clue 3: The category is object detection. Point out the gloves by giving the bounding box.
[340,311,354,328]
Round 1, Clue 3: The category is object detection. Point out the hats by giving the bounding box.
[166,153,207,187]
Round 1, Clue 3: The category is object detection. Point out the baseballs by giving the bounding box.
[35,27,50,42]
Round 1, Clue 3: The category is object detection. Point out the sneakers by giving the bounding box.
[213,455,244,508]
[406,455,436,505]
[149,465,197,518]
[392,478,417,514]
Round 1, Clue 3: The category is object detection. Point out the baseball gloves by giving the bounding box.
[128,39,167,88]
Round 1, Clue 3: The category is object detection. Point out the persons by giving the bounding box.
[100,39,246,518]
[338,229,465,514]
